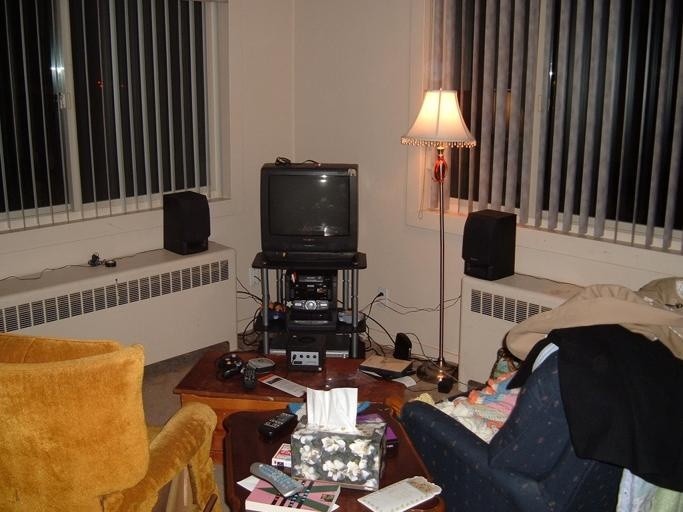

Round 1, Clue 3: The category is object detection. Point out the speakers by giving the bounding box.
[163,191,212,256]
[460,209,517,282]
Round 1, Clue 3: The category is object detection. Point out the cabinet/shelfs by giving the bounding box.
[251,251,367,360]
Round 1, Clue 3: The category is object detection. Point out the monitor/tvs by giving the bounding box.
[261,162,358,263]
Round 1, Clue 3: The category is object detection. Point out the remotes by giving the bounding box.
[250,461,303,500]
[243,366,259,390]
[259,413,296,442]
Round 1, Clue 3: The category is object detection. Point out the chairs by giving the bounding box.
[0,330,219,510]
[399,346,626,512]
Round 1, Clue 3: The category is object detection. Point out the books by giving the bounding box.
[355,412,401,450]
[359,353,413,376]
[243,476,341,512]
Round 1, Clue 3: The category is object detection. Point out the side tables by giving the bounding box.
[220,401,443,511]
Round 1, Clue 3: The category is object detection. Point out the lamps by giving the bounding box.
[399,89,477,383]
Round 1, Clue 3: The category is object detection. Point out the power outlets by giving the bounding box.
[376,287,387,308]
